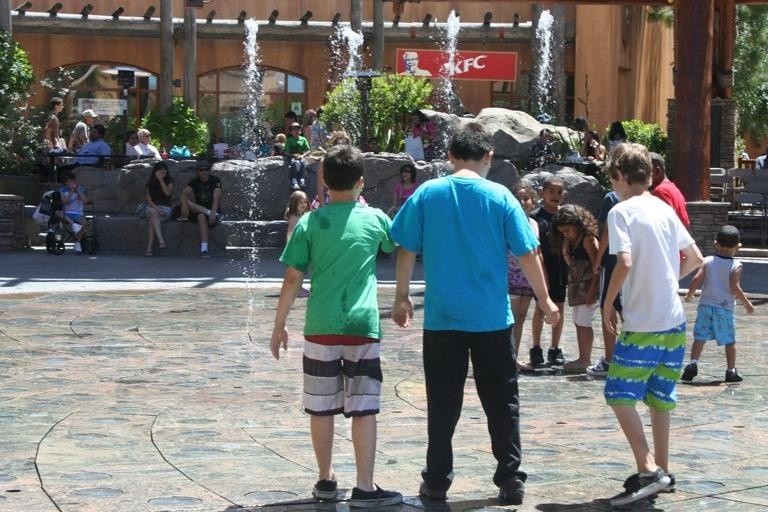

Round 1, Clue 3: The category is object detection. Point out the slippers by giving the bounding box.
[563,361,587,374]
[158,242,168,250]
[144,250,153,258]
[516,364,534,373]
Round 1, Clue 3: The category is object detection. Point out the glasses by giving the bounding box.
[196,168,210,171]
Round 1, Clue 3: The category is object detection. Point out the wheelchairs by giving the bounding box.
[45,199,98,255]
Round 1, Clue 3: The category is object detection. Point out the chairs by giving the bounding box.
[733,157,764,215]
[710,165,725,200]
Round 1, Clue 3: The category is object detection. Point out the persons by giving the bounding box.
[271,144,402,508]
[586,189,625,377]
[650,151,691,227]
[601,143,703,507]
[39,97,163,163]
[54,172,90,252]
[680,225,755,381]
[399,49,434,76]
[144,160,173,254]
[366,109,435,262]
[508,177,600,375]
[267,107,367,296]
[526,119,627,171]
[389,125,562,504]
[177,159,222,259]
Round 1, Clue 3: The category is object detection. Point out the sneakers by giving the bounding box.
[349,483,404,509]
[74,242,82,252]
[609,466,672,508]
[529,347,545,364]
[70,222,82,233]
[498,478,525,506]
[585,355,611,376]
[726,368,744,383]
[200,250,210,259]
[299,178,307,187]
[312,478,338,501]
[215,212,225,223]
[547,347,566,365]
[658,470,677,494]
[681,362,698,381]
[288,178,301,190]
[418,484,448,504]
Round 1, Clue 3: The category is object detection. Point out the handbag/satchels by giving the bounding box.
[136,203,149,219]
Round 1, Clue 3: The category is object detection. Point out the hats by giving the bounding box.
[81,109,98,119]
[195,160,210,170]
